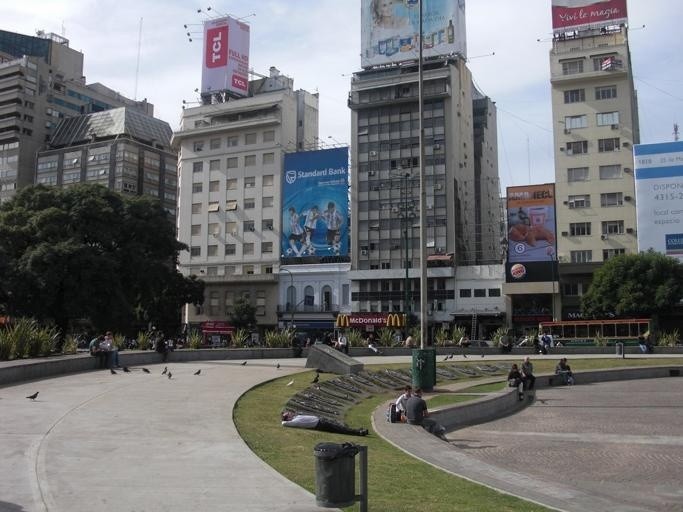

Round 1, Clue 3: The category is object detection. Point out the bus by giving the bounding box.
[538,319,657,346]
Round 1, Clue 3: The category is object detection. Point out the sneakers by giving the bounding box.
[357,426,369,436]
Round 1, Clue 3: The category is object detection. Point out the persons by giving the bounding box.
[366,333,384,356]
[405,387,442,439]
[555,358,568,385]
[98,331,121,368]
[88,335,107,369]
[518,207,530,227]
[320,202,344,256]
[294,206,320,257]
[368,1,408,50]
[327,334,338,351]
[454,334,469,346]
[395,385,413,416]
[520,356,535,391]
[405,333,417,349]
[518,335,529,347]
[154,330,169,363]
[281,410,368,436]
[563,358,576,385]
[286,207,315,257]
[336,331,347,355]
[291,334,303,358]
[298,331,331,349]
[644,334,653,353]
[507,363,524,400]
[507,334,513,352]
[541,332,551,353]
[498,333,514,354]
[638,332,647,354]
[533,336,541,353]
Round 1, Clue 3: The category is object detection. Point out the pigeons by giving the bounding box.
[240,362,247,366]
[286,378,295,387]
[110,366,172,379]
[442,356,448,361]
[464,354,467,358]
[25,391,39,400]
[277,363,280,368]
[309,373,319,384]
[194,370,201,375]
[449,353,453,358]
[481,353,484,357]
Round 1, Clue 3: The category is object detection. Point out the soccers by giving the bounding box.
[306,247,315,254]
[331,244,340,253]
[285,248,293,256]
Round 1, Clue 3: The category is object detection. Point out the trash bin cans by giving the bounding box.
[616,343,622,354]
[314,441,356,508]
[412,349,436,393]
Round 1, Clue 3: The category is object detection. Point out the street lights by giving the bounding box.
[279,268,293,329]
